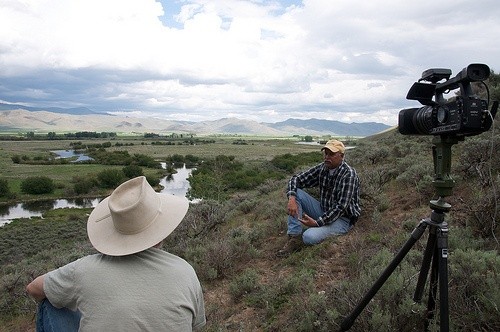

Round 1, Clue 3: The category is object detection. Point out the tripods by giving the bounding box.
[340,139,461,332]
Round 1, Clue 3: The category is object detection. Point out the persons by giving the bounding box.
[26,176,207,331]
[277,139,362,256]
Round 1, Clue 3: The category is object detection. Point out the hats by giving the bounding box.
[87,175,189,256]
[321,139,346,154]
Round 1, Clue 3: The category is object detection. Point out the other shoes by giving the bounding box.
[277,236,304,256]
